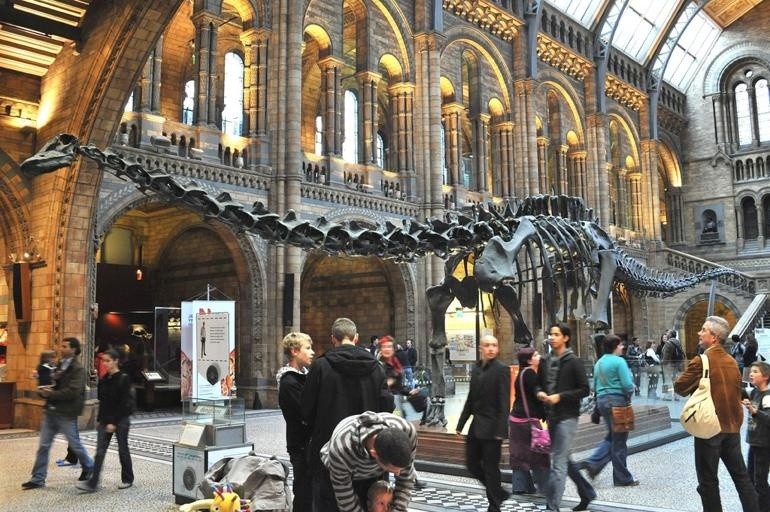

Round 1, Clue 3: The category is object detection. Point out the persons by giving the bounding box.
[645,340,661,400]
[574,334,648,487]
[743,333,759,367]
[276,331,316,511]
[20,336,94,490]
[314,410,416,512]
[510,348,551,495]
[703,215,716,232]
[200,322,207,357]
[377,335,403,377]
[416,365,434,388]
[627,338,641,397]
[94,339,113,382]
[731,334,747,373]
[666,329,670,335]
[456,334,513,512]
[673,315,758,512]
[696,342,706,355]
[661,330,686,403]
[36,349,63,387]
[56,441,77,467]
[741,362,770,512]
[75,350,137,490]
[368,336,378,353]
[529,323,596,512]
[300,317,396,511]
[365,479,396,510]
[656,334,668,395]
[406,338,416,363]
[365,480,393,512]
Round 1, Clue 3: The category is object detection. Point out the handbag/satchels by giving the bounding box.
[519,367,552,456]
[681,354,721,441]
[597,362,637,433]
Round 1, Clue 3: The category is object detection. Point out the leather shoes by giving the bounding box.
[58,460,73,467]
[75,483,98,492]
[573,494,595,511]
[118,482,132,488]
[20,481,45,489]
[79,467,95,480]
[56,458,63,463]
[614,478,641,487]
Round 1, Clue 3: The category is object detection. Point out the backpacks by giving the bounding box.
[668,340,685,363]
[116,373,136,417]
[100,373,136,419]
